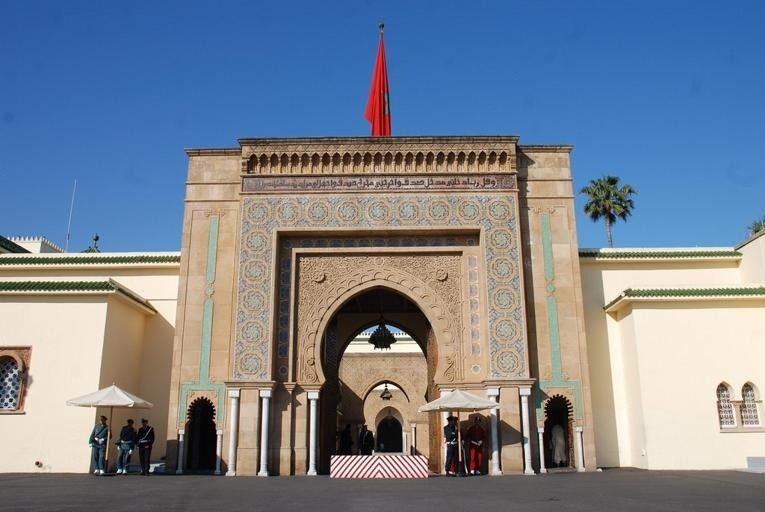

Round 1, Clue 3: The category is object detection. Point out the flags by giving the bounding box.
[363,35,391,138]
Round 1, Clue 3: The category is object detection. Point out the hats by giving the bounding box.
[447,415,482,422]
[100,415,149,422]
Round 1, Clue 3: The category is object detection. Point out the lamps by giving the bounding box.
[381,382,392,401]
[367,314,396,350]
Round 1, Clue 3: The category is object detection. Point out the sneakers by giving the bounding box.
[446,470,452,477]
[140,471,150,476]
[93,469,105,474]
[470,470,481,475]
[116,469,128,475]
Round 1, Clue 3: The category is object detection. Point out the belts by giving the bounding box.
[121,439,152,444]
[95,437,105,442]
[446,440,483,445]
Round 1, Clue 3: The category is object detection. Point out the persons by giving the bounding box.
[358,424,375,455]
[465,417,487,476]
[442,415,462,477]
[115,419,137,474]
[551,421,566,467]
[87,414,112,476]
[338,424,355,455]
[135,417,155,476]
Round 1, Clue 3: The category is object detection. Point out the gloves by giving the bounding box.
[129,449,133,455]
[116,446,121,451]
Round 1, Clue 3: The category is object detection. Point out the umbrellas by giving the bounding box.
[66,386,155,471]
[415,388,503,473]
